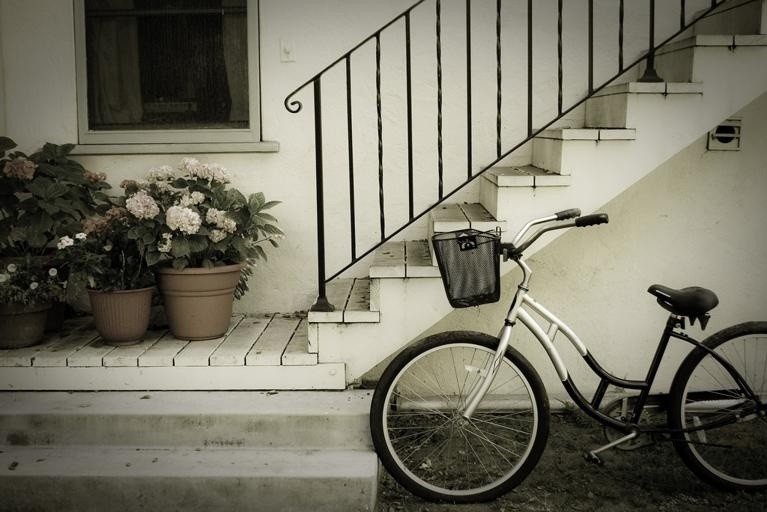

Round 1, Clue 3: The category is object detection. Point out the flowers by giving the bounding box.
[115,159,281,267]
[2,136,104,256]
[59,206,154,292]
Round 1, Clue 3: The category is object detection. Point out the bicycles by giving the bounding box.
[369,208,766,503]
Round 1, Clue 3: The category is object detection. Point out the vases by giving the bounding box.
[1,260,246,349]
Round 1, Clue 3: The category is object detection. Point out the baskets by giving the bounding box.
[431,228,501,309]
